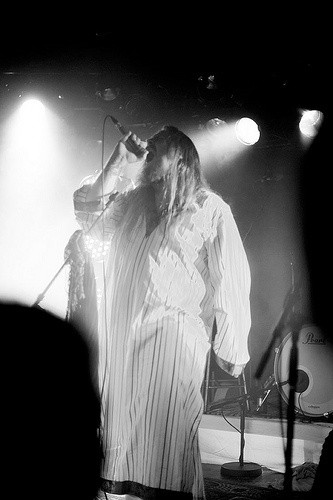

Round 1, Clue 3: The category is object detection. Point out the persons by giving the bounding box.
[63,121,253,500]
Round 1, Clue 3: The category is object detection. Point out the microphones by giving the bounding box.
[108,115,147,159]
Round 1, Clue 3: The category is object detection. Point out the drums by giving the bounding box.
[275,325,333,417]
